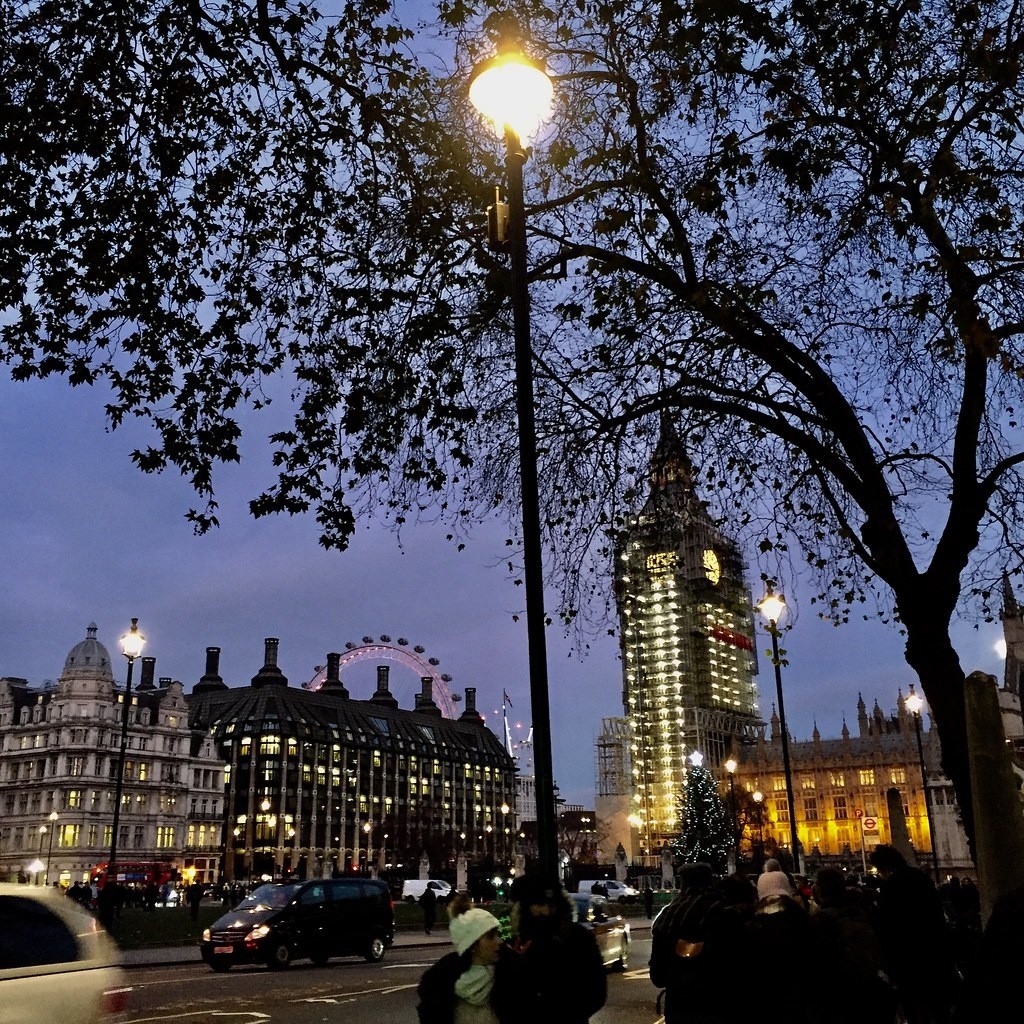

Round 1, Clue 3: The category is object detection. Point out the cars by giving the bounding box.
[0,883,127,1023]
[566,893,631,971]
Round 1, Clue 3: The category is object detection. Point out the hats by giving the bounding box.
[449,908,500,956]
[510,873,573,945]
[757,858,792,901]
[677,863,712,890]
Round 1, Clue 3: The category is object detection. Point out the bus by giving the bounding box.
[90,861,171,887]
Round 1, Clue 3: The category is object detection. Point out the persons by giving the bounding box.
[419,882,437,934]
[179,879,204,920]
[643,885,654,919]
[445,884,460,906]
[648,844,982,1024]
[212,882,258,905]
[414,871,608,1024]
[162,884,169,909]
[591,881,611,899]
[53,880,157,914]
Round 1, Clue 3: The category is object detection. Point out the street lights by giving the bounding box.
[364,823,371,876]
[724,754,738,872]
[468,36,560,905]
[904,684,939,886]
[45,812,59,884]
[101,618,146,933]
[755,581,800,873]
[34,826,47,884]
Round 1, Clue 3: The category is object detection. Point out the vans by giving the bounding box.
[402,880,460,904]
[579,880,640,904]
[201,876,395,972]
